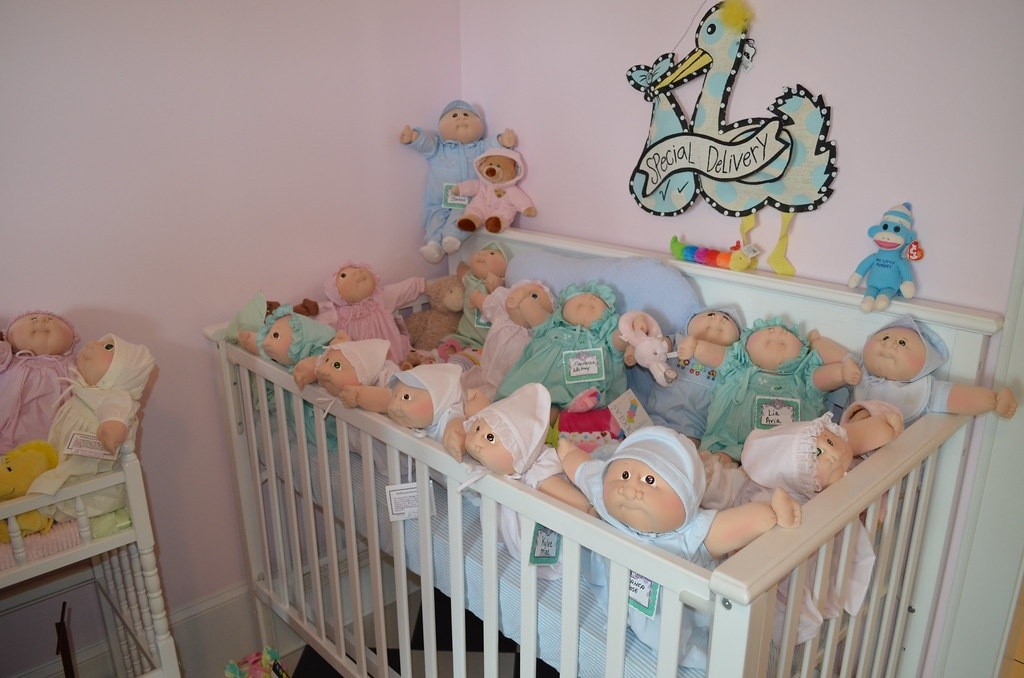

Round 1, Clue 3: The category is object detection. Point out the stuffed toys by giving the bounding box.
[848,201,918,315]
[669,235,762,271]
[397,99,537,264]
[0,311,156,547]
[231,245,1017,661]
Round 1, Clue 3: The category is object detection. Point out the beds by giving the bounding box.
[0,449,183,678]
[205,231,1003,678]
